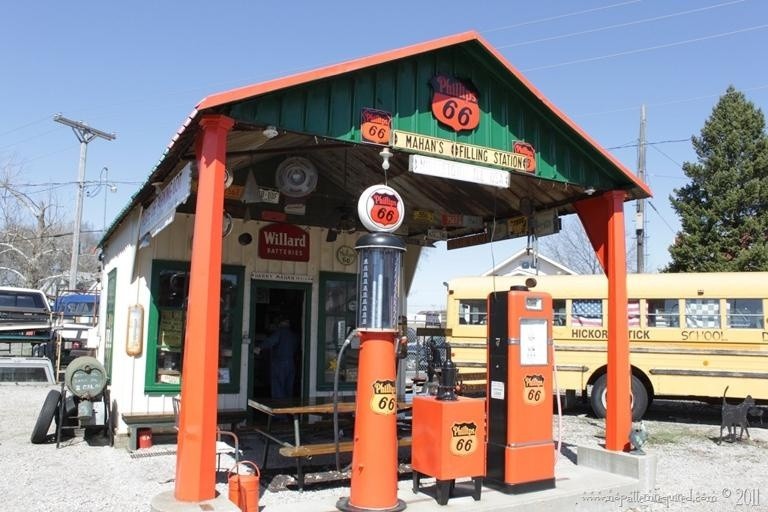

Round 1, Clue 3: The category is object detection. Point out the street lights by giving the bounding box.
[56,113,114,294]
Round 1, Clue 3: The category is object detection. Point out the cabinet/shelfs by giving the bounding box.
[583,187,596,196]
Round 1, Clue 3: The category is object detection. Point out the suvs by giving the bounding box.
[1,286,51,356]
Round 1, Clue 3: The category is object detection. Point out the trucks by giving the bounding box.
[52,296,101,364]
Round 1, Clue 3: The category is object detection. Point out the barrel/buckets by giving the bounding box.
[137,427,153,449]
[228,460,261,512]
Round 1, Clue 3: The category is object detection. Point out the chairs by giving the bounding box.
[170,395,240,483]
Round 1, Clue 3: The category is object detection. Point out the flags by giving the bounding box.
[663,299,731,329]
[571,300,647,328]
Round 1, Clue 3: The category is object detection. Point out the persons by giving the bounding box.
[395,335,408,379]
[425,341,442,366]
[252,318,298,426]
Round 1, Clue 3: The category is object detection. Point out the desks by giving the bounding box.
[245,393,414,493]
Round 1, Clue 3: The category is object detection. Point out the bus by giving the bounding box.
[445,272,768,421]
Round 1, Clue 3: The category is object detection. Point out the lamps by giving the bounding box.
[151,181,164,194]
[377,147,395,171]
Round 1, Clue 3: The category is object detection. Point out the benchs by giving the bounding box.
[121,407,247,450]
[278,435,412,457]
